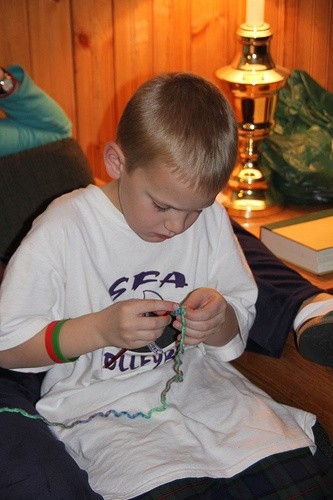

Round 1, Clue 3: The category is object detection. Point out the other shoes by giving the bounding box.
[292,292,332,367]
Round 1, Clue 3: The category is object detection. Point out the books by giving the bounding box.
[259,210,333,275]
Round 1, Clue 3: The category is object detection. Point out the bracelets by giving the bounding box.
[45,317,80,365]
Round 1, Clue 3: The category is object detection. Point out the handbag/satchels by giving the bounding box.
[257,69,333,209]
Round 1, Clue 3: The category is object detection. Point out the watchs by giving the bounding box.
[0,66,13,96]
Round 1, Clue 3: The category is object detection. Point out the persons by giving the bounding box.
[0,69,328,500]
[0,65,333,365]
